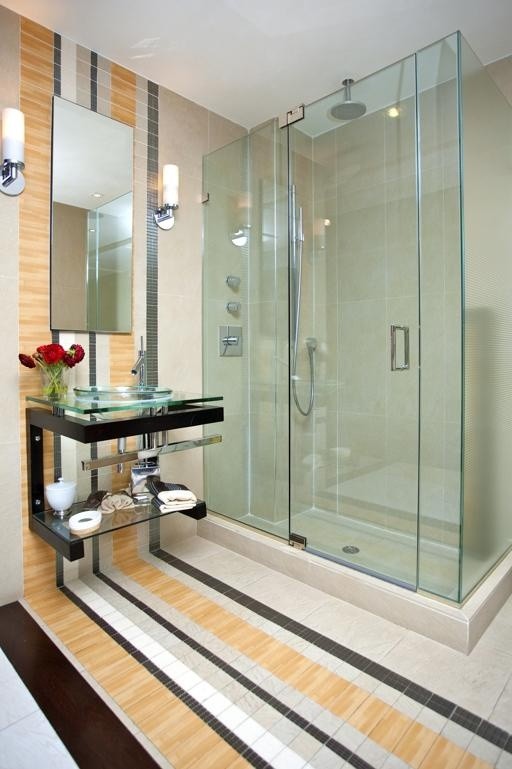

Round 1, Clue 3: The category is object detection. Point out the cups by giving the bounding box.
[45,478,76,517]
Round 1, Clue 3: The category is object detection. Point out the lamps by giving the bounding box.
[0,109,26,195]
[154,164,180,229]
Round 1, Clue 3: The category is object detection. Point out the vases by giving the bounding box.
[41,365,66,400]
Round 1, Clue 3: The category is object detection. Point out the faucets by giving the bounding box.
[129,334,149,389]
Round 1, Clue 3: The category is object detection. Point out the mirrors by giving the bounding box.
[51,94,131,334]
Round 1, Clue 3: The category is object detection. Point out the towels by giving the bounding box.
[150,481,195,515]
[82,486,136,514]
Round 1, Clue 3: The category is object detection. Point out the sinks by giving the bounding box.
[73,384,173,419]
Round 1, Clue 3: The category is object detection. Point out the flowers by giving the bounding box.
[18,341,83,371]
